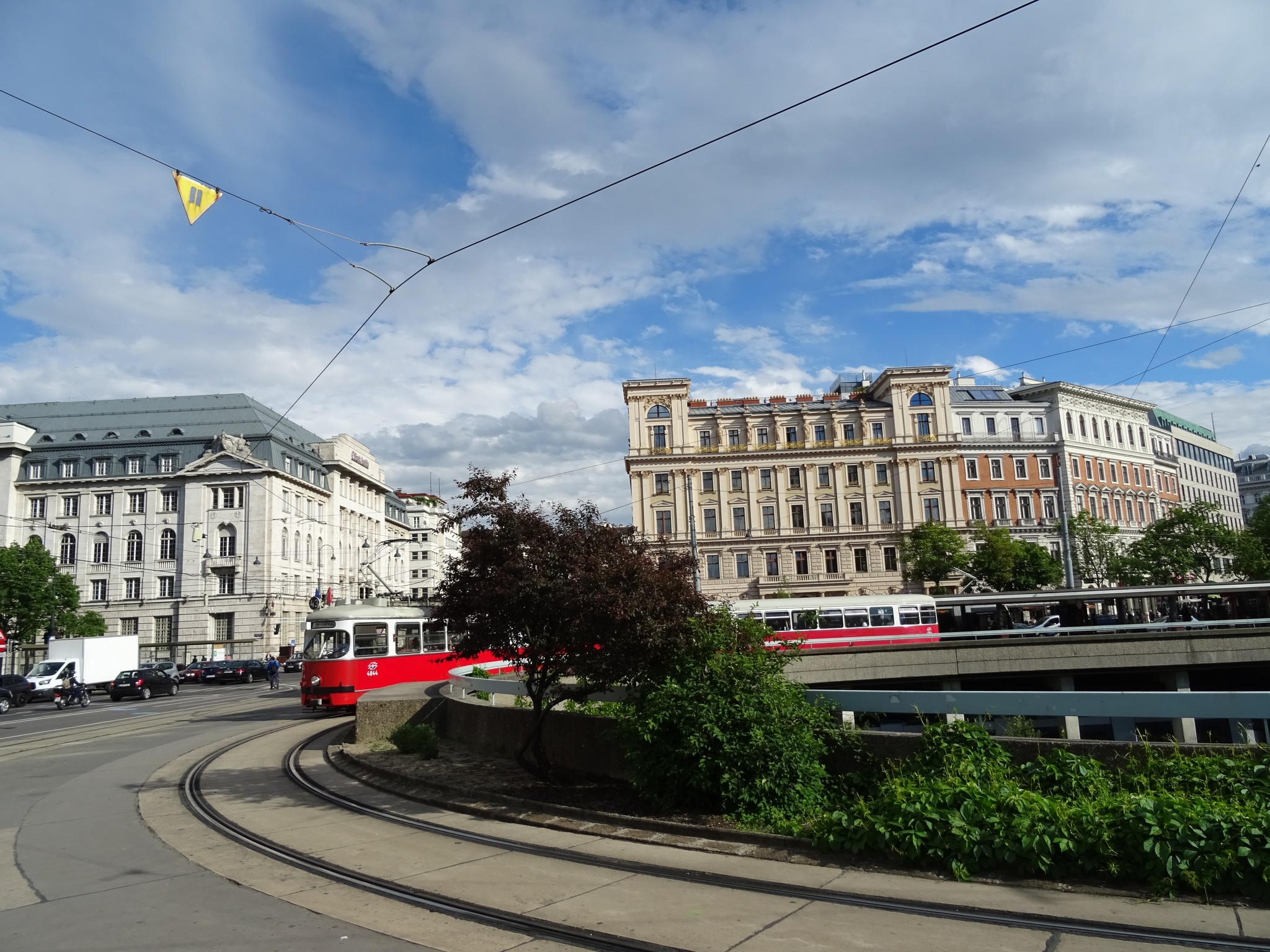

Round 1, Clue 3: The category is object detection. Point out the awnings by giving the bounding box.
[1207,594,1222,599]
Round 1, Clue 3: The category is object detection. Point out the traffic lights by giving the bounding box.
[9,640,22,653]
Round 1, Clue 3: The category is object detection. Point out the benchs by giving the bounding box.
[123,672,138,678]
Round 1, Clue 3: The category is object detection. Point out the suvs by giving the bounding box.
[139,661,179,684]
[0,674,32,714]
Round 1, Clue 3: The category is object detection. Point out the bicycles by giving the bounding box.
[257,658,284,669]
[272,673,280,690]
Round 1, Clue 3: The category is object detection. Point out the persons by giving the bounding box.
[266,651,281,690]
[1031,616,1036,625]
[412,634,459,652]
[771,616,868,631]
[63,670,86,703]
[201,655,207,661]
[1127,610,1161,624]
[339,636,358,653]
[193,655,197,663]
[1166,602,1231,631]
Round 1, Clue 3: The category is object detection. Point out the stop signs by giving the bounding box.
[0,640,6,652]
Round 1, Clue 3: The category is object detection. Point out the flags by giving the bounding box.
[325,587,333,605]
[315,588,321,598]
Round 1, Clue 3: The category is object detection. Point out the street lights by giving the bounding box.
[318,545,336,655]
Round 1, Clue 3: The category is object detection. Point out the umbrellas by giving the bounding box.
[1156,598,1213,604]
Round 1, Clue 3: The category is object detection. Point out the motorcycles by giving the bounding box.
[52,679,91,710]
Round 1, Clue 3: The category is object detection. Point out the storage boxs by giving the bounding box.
[53,685,66,693]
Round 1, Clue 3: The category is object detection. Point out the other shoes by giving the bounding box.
[270,688,273,689]
[277,678,281,681]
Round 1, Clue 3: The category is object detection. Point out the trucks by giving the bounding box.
[24,634,139,702]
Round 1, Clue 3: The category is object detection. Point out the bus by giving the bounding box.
[300,537,940,716]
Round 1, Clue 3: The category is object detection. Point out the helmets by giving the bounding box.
[67,670,75,674]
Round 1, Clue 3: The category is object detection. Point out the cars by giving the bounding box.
[178,659,271,684]
[1087,614,1140,628]
[1146,615,1207,632]
[110,668,179,700]
[283,651,325,673]
[992,615,1061,638]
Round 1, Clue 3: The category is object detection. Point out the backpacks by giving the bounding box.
[270,661,278,674]
[61,677,73,689]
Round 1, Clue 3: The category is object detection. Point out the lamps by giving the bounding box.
[253,556,261,567]
[203,549,212,561]
[361,539,370,551]
[744,529,753,541]
[268,611,275,616]
[274,624,281,634]
[1037,517,1046,530]
[394,549,401,560]
